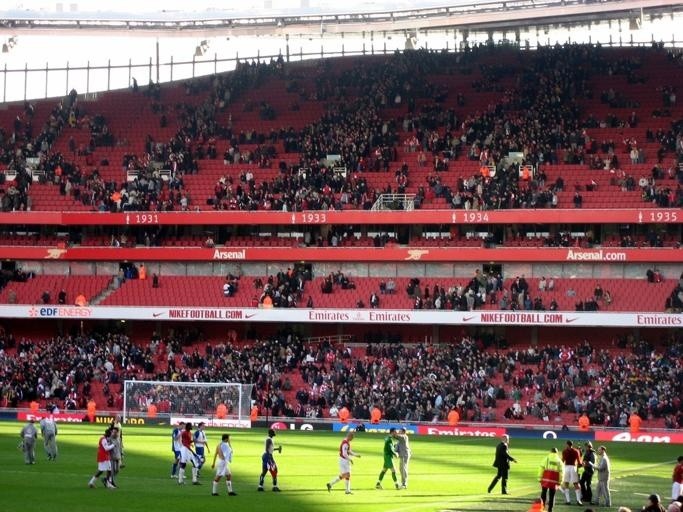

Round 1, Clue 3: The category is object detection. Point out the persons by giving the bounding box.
[536,447,563,512]
[376,427,401,490]
[39,415,57,461]
[111,414,126,469]
[559,439,683,512]
[257,429,282,492]
[326,432,361,495]
[487,434,519,495]
[88,429,117,488]
[393,427,411,489]
[171,421,238,496]
[20,420,37,465]
[102,428,119,485]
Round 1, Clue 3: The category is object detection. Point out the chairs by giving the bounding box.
[0,332,60,357]
[0,229,681,249]
[128,338,256,380]
[475,348,668,427]
[0,52,682,212]
[312,341,367,366]
[273,368,313,413]
[16,381,124,411]
[0,274,683,314]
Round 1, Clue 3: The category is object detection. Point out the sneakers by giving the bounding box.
[487,488,509,494]
[87,475,119,490]
[375,484,383,489]
[212,486,281,496]
[326,483,333,493]
[168,474,202,486]
[345,491,354,495]
[394,483,407,490]
[565,495,612,507]
